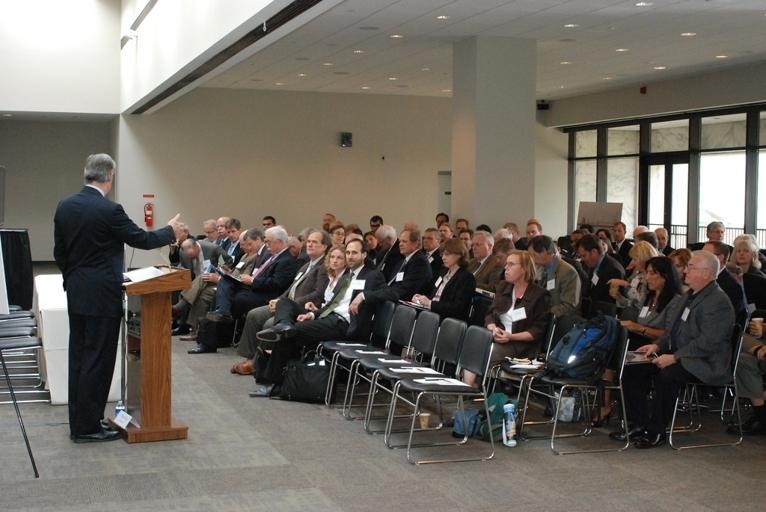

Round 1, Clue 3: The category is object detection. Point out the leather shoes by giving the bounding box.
[231,360,256,375]
[171,326,189,335]
[248,383,276,398]
[633,432,667,449]
[203,309,235,325]
[179,335,197,341]
[99,420,113,430]
[254,323,296,343]
[172,304,181,319]
[74,428,122,444]
[609,426,646,441]
[187,342,217,354]
[727,414,766,436]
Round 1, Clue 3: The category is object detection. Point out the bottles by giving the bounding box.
[114,399,124,417]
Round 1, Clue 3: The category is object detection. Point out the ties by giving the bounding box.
[387,258,410,287]
[470,261,481,275]
[227,243,235,256]
[315,271,356,320]
[251,254,276,280]
[286,261,314,302]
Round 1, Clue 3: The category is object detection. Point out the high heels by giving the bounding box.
[590,406,613,428]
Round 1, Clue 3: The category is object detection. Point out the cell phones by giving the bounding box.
[652,353,658,359]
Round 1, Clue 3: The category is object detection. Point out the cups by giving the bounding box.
[400,346,414,368]
[753,317,764,323]
[419,413,431,429]
[207,264,215,272]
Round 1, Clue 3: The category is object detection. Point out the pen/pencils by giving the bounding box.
[653,352,658,358]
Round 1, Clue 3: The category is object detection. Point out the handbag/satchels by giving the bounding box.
[479,391,520,443]
[278,349,338,407]
[451,407,482,437]
[545,388,583,424]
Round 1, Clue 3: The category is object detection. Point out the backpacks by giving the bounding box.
[544,314,621,386]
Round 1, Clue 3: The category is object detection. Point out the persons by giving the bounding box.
[54,152,185,444]
[171,214,436,398]
[412,213,764,449]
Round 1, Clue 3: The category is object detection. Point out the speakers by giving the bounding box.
[343,133,352,147]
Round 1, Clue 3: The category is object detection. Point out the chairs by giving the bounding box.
[0,302,50,405]
[207,258,766,469]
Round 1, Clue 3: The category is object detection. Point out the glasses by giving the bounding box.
[683,263,707,272]
[503,261,521,266]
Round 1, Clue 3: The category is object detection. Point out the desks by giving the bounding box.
[31,270,132,407]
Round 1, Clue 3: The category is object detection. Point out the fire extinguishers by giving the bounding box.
[143,203,154,228]
